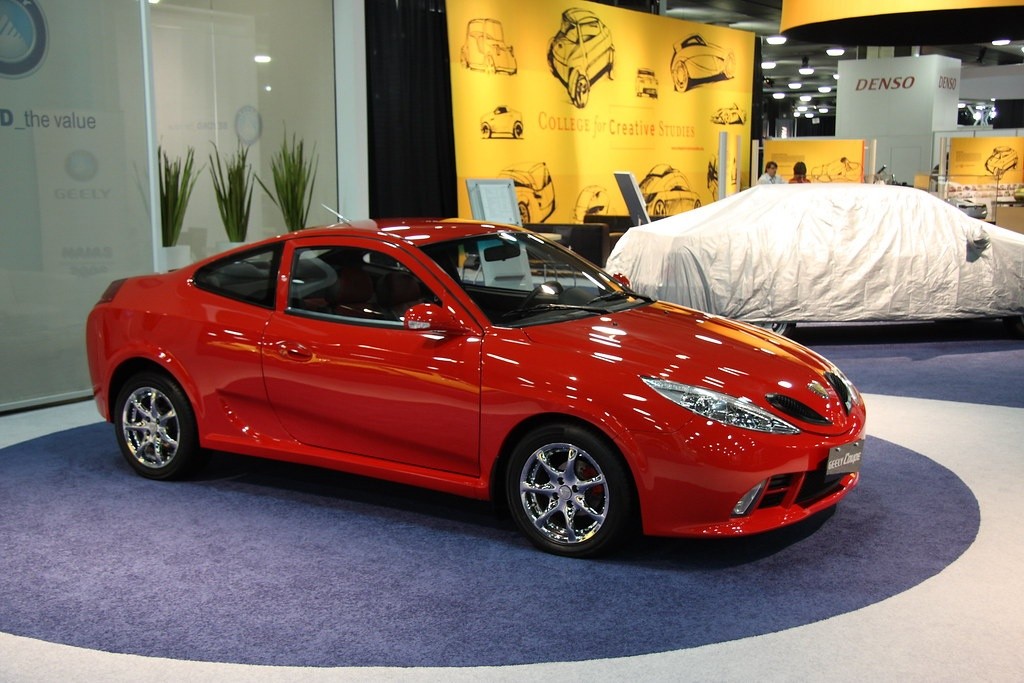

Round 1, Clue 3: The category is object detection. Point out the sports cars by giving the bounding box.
[84,219,865,556]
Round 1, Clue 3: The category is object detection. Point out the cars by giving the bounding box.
[596,180,1022,338]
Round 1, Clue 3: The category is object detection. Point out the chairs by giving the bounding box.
[380,272,433,322]
[337,273,378,319]
[461,243,481,284]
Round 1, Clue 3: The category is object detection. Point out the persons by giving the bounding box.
[789,162,811,183]
[757,162,787,184]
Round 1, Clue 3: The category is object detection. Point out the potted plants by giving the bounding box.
[157,144,208,272]
[207,133,258,251]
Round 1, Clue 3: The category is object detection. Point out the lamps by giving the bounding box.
[826,48,845,57]
[773,93,785,100]
[799,96,812,102]
[799,56,815,74]
[788,83,802,89]
[766,36,787,45]
[818,87,831,93]
[761,62,776,69]
